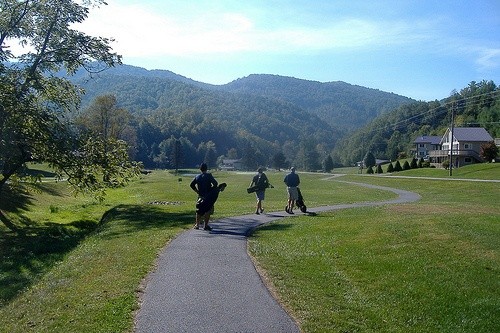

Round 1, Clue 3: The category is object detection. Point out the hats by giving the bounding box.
[200,163,207,170]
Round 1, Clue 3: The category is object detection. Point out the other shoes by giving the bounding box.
[260,208,264,213]
[194,225,199,229]
[255,211,260,215]
[287,209,294,214]
[203,226,213,231]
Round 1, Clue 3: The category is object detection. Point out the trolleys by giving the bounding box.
[297,186,307,212]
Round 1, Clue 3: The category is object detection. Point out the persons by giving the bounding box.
[249,168,269,215]
[190,163,218,230]
[284,167,300,214]
